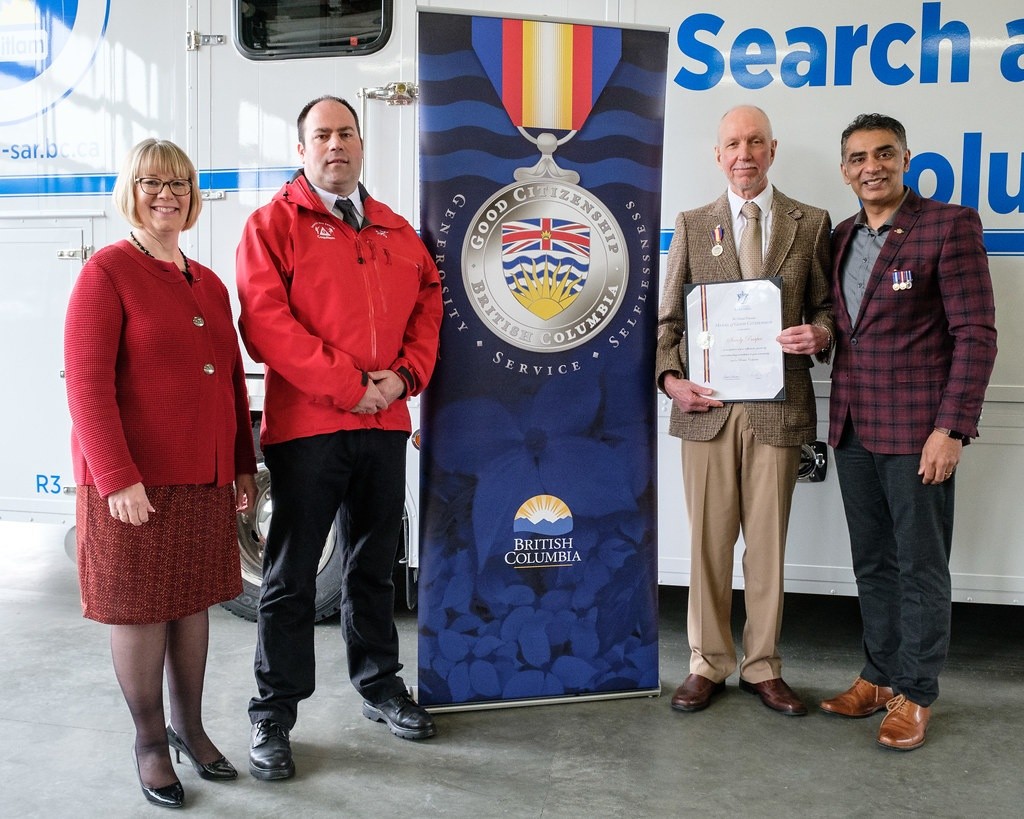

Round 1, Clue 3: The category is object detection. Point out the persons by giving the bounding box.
[63,138,259,808]
[654,105,837,717]
[817,113,999,752]
[248,95,444,781]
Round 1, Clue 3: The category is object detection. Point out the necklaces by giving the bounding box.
[130,231,191,281]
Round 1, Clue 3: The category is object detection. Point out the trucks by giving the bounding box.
[0,0,1024,624]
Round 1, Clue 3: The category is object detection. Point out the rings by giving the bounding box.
[944,472,953,475]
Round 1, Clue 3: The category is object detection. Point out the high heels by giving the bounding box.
[131,740,185,807]
[166,722,239,780]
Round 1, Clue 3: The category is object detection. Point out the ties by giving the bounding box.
[334,198,360,233]
[737,202,763,281]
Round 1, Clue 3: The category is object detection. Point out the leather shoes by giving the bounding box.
[248,717,296,780]
[738,675,808,716]
[819,676,895,718]
[362,690,438,739]
[875,693,932,752]
[670,673,726,711]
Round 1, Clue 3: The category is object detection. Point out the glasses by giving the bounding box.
[135,177,192,196]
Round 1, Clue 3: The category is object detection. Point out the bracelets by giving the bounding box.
[815,325,831,352]
[933,426,965,441]
